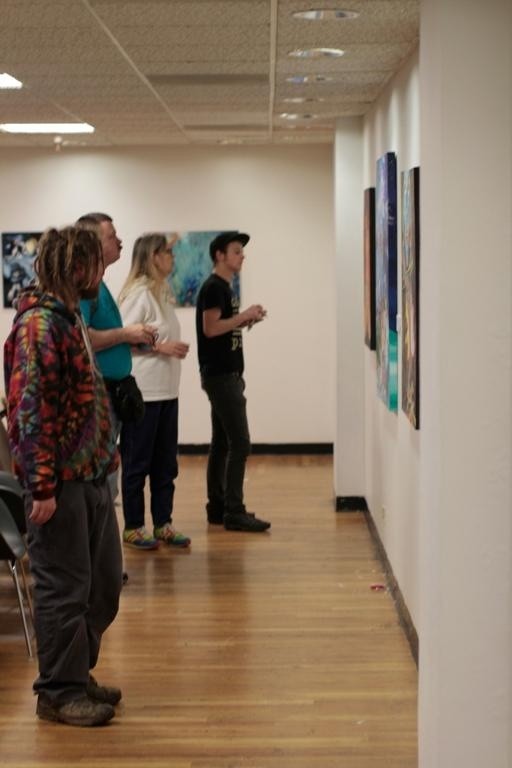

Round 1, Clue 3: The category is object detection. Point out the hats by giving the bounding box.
[209,231,250,257]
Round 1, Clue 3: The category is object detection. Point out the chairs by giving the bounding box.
[1,502,42,666]
[0,470,40,639]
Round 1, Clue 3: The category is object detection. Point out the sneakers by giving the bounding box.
[122,526,161,551]
[152,522,191,549]
[207,508,255,525]
[36,692,115,727]
[81,674,122,706]
[224,513,270,533]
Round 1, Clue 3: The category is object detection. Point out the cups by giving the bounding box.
[138,325,158,353]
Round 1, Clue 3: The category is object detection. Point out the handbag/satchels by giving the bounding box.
[102,373,148,424]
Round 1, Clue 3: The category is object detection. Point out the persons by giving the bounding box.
[69,212,137,582]
[195,232,272,535]
[2,225,128,727]
[118,231,188,551]
